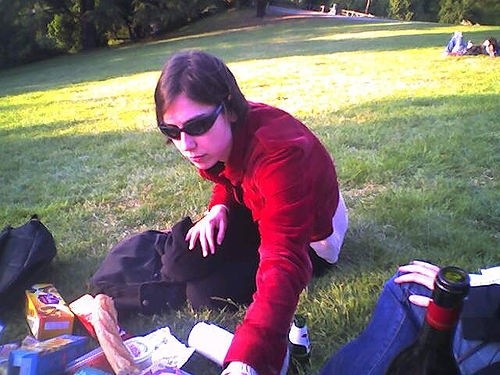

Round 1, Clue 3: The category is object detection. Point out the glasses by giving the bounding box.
[158,104,225,139]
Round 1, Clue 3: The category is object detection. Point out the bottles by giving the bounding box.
[289,314,313,375]
[386,266,471,375]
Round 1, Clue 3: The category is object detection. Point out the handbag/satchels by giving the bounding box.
[92,216,194,312]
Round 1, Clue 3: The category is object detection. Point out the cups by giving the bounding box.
[124,336,154,370]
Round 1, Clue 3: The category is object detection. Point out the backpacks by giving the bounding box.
[0,213,57,313]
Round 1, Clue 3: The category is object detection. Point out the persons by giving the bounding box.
[319,260,500,375]
[154,50,348,375]
[447,34,497,54]
